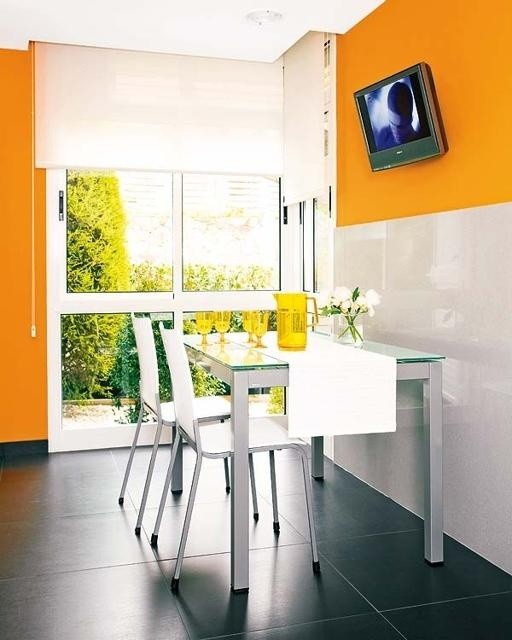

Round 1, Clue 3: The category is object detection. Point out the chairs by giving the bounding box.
[156,320,321,592]
[121,311,259,534]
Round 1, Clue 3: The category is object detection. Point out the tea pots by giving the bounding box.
[273,292,319,352]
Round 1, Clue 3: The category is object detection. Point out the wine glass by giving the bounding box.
[243,310,270,349]
[196,311,231,348]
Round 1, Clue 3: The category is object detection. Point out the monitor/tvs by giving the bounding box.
[352,61,449,172]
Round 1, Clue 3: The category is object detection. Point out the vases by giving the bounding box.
[330,314,364,341]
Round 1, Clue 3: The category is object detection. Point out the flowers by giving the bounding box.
[319,284,380,342]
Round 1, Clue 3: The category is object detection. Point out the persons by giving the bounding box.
[376,81,419,148]
